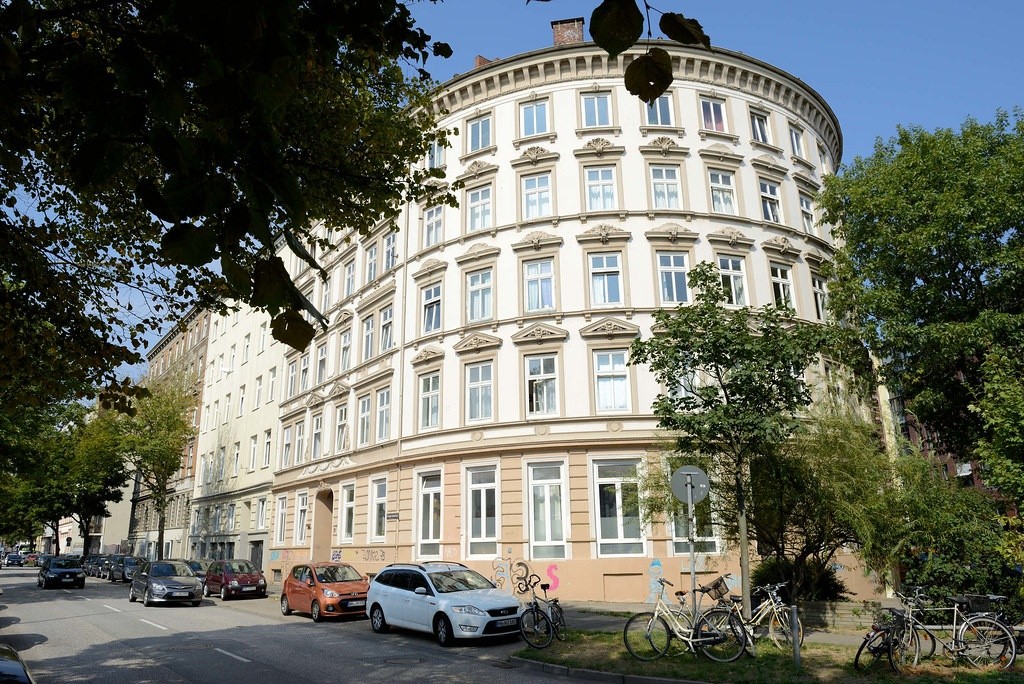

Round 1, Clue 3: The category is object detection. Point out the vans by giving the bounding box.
[19,551,41,559]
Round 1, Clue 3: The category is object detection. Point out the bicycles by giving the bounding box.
[624,572,803,662]
[518,574,567,648]
[854,585,1024,672]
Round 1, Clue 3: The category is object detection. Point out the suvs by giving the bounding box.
[100,553,130,580]
[364,561,527,646]
[84,554,102,575]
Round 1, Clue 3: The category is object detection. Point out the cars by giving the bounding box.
[110,557,148,583]
[64,554,81,567]
[90,556,108,577]
[203,559,267,601]
[129,560,202,608]
[4,554,24,567]
[25,554,40,564]
[280,563,371,623]
[36,555,54,567]
[0,643,36,684]
[182,559,215,584]
[79,555,87,570]
[36,558,86,590]
